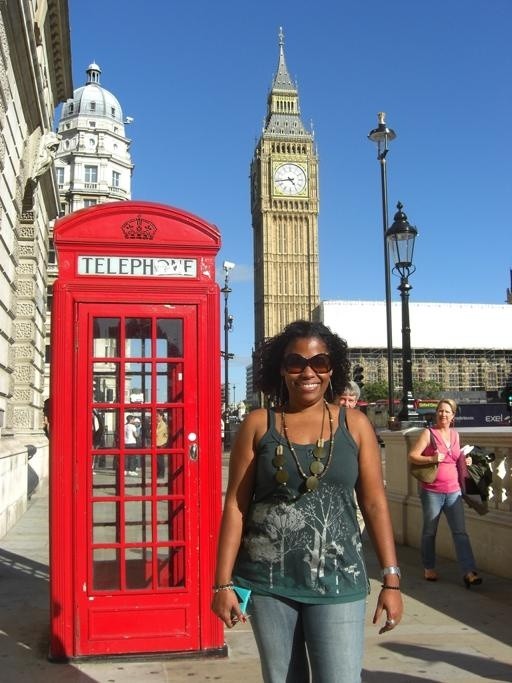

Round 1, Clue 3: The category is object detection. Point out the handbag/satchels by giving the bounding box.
[410,463,438,484]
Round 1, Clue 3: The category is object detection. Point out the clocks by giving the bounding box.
[274,162,308,196]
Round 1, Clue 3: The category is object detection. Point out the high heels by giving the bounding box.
[463,573,483,585]
[422,568,437,580]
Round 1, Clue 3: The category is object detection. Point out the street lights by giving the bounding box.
[216,260,235,453]
[365,108,398,429]
[382,197,427,423]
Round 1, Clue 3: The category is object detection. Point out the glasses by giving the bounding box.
[281,352,336,374]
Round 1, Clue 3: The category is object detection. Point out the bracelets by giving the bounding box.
[380,584,400,590]
[213,581,234,594]
[380,566,401,579]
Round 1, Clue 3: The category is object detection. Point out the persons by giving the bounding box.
[332,379,368,543]
[407,397,483,589]
[154,408,169,479]
[42,397,52,441]
[209,314,405,681]
[124,413,142,477]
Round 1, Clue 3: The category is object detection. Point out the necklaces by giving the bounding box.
[272,394,334,503]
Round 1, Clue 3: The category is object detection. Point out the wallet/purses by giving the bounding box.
[231,584,252,618]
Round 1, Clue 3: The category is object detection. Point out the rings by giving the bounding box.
[386,620,395,624]
